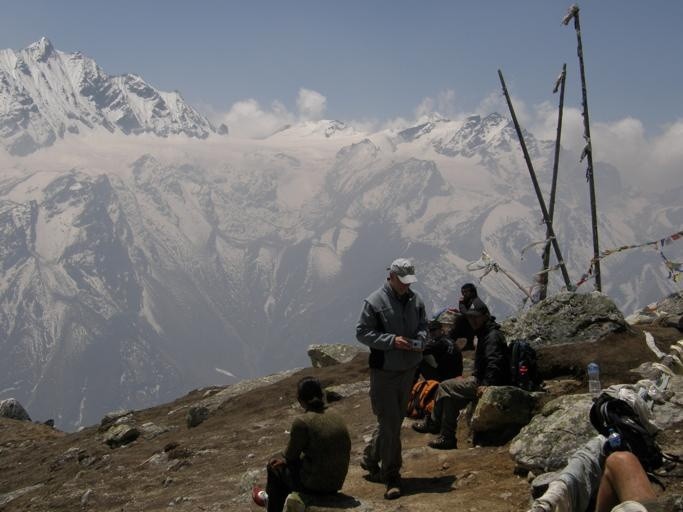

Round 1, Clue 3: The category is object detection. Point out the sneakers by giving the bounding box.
[361,462,381,475]
[252,487,267,506]
[384,483,401,500]
[412,415,441,434]
[427,435,457,449]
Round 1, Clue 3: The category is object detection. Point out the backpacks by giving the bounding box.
[405,380,440,419]
[506,339,539,392]
[590,392,662,472]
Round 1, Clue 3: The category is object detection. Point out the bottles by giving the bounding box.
[608,428,621,451]
[587,359,601,394]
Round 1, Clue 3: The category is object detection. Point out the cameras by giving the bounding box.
[411,340,422,349]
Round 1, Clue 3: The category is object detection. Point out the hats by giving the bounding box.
[428,321,442,330]
[391,258,418,284]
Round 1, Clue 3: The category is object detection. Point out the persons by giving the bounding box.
[595,448,662,512]
[356,257,429,499]
[250,375,351,512]
[447,282,492,351]
[410,299,509,450]
[410,320,463,389]
